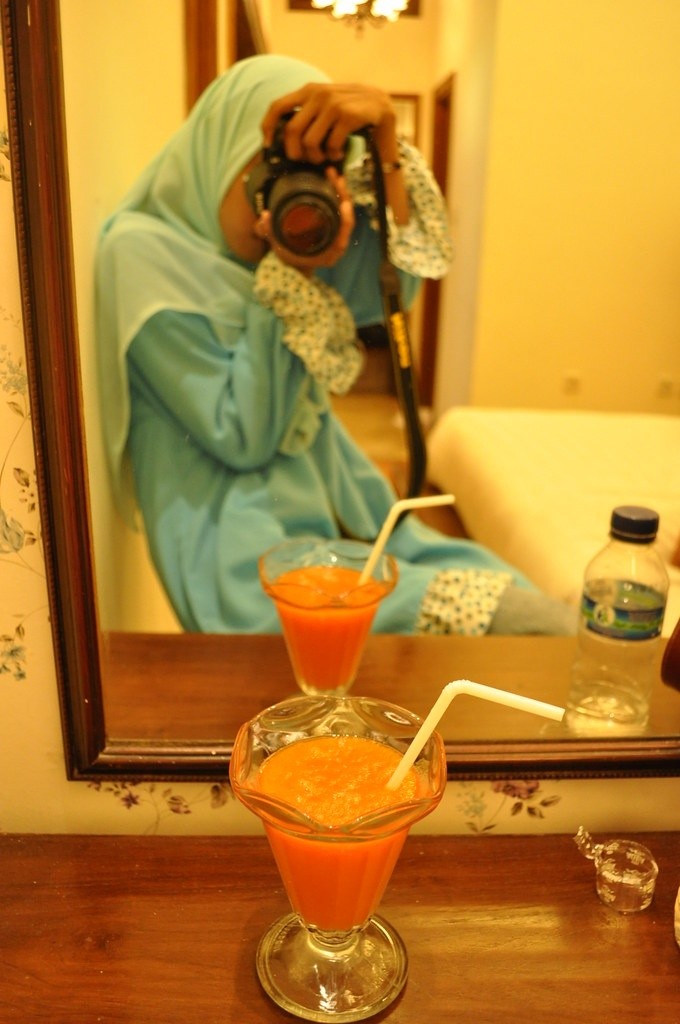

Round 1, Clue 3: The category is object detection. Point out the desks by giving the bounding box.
[99,629,680,739]
[0,830,680,1024]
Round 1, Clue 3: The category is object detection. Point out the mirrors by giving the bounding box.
[0,0,680,784]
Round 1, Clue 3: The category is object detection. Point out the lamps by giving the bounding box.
[312,0,409,43]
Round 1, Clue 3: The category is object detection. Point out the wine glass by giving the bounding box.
[228,695,447,1024]
[259,535,398,694]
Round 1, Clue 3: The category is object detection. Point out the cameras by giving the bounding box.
[244,110,351,256]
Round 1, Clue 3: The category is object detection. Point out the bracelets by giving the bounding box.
[381,157,405,173]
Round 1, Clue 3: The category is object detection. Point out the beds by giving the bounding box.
[425,404,680,637]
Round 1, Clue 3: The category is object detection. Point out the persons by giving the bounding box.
[93,53,579,634]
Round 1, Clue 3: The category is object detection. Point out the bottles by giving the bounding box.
[565,504,671,738]
